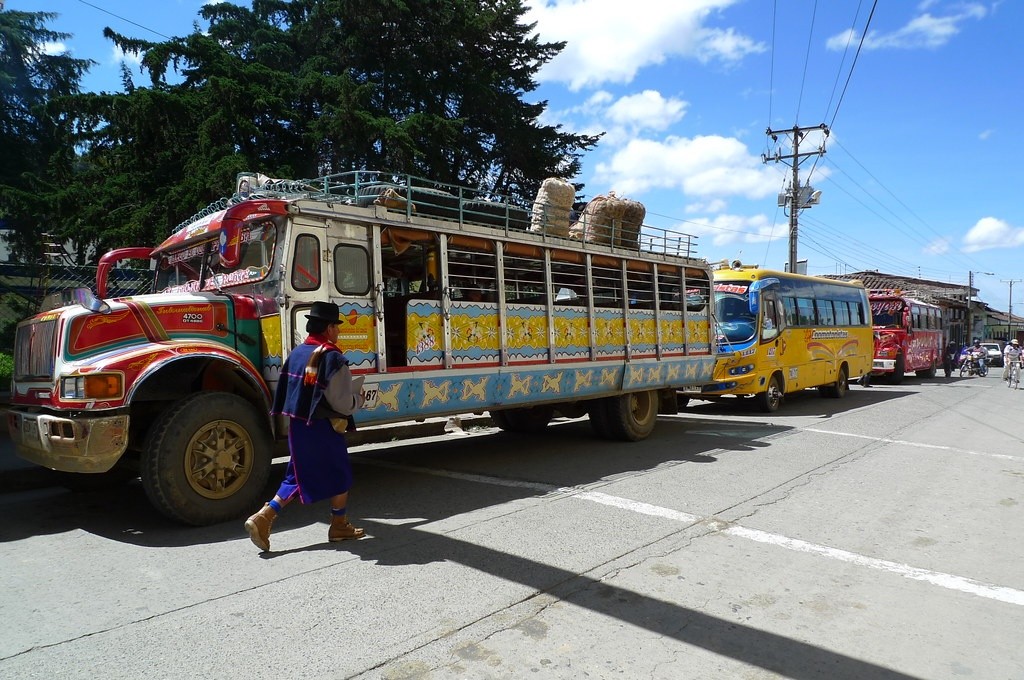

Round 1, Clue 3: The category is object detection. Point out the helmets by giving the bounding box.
[973,340,980,347]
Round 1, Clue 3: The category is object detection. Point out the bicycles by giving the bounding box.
[1006,358,1022,389]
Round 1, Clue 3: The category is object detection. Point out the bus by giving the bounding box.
[677,258,875,413]
[0,171,715,527]
[863,288,944,384]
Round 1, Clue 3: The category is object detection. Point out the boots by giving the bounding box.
[329,513,366,540]
[245,502,276,551]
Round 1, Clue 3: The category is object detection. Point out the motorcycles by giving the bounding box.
[960,350,987,378]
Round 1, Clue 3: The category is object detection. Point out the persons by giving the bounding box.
[244,301,366,552]
[947,340,987,374]
[1001,339,1024,383]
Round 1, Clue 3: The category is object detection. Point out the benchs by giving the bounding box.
[385,290,441,347]
[507,297,706,311]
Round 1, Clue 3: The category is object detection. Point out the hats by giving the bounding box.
[304,301,343,324]
[1011,338,1019,345]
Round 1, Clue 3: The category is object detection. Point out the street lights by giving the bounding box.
[967,270,994,347]
[790,190,822,274]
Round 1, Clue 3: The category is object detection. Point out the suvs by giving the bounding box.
[980,343,1002,367]
